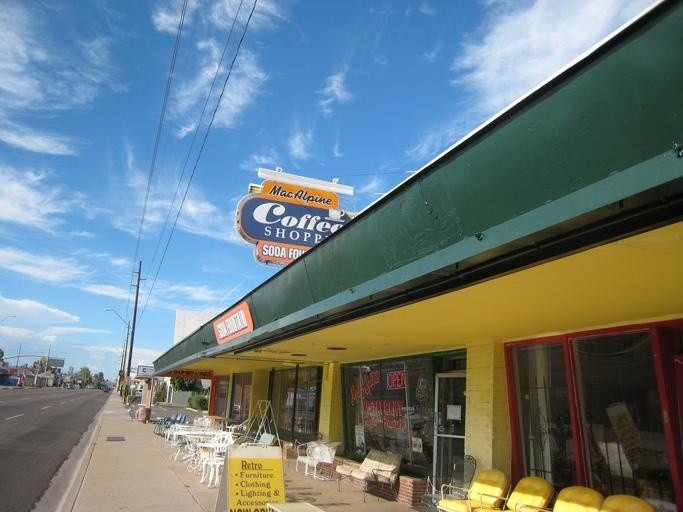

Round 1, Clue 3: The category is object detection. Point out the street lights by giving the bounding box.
[0,315,17,325]
[103,308,130,391]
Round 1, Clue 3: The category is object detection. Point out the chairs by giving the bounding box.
[151,398,281,487]
[294,439,403,504]
[424,453,661,511]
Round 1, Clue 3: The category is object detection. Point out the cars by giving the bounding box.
[134,388,142,397]
[99,385,112,393]
[233,405,240,414]
